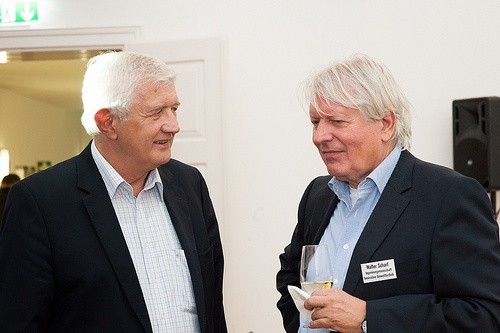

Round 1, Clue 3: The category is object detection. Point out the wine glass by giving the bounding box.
[300,246,334,331]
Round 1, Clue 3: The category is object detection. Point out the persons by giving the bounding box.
[0,173,20,218]
[276,54,500,333]
[2,50,228,333]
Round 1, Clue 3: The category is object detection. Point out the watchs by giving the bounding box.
[360,319,368,332]
